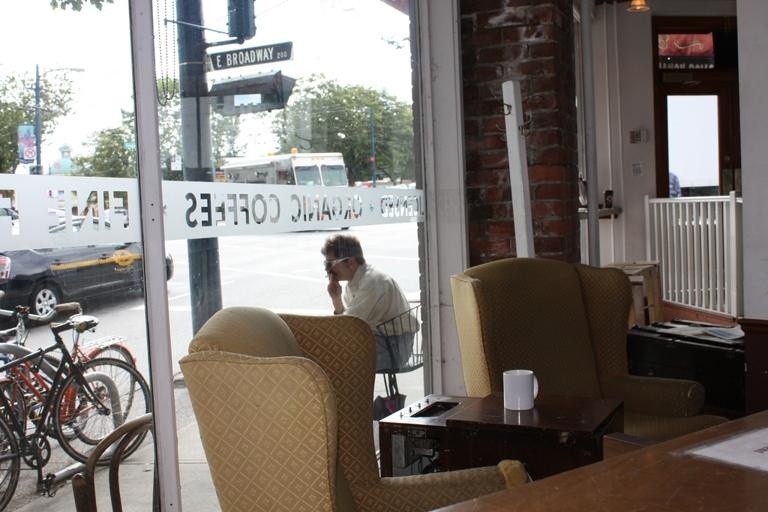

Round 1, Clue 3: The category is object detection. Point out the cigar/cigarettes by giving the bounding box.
[323,274,329,278]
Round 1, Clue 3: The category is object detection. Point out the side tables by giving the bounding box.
[377,395,625,479]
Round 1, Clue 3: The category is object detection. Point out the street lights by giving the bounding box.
[36,64,84,175]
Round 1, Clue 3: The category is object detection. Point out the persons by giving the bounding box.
[321,236,419,373]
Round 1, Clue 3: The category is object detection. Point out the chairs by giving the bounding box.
[375,300,424,409]
[448,256,729,458]
[178,306,533,512]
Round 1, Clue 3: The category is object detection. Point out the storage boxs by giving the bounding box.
[603,259,663,329]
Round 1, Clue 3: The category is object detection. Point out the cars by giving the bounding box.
[353,183,416,218]
[0,209,174,324]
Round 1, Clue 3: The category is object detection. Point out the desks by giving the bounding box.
[433,405,767,512]
[626,319,767,419]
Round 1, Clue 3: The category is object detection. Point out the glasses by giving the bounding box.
[324,256,350,273]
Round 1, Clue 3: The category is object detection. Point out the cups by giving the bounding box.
[503,369,539,411]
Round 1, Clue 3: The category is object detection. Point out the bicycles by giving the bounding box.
[0,302,151,512]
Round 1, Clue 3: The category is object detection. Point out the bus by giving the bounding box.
[220,148,351,229]
